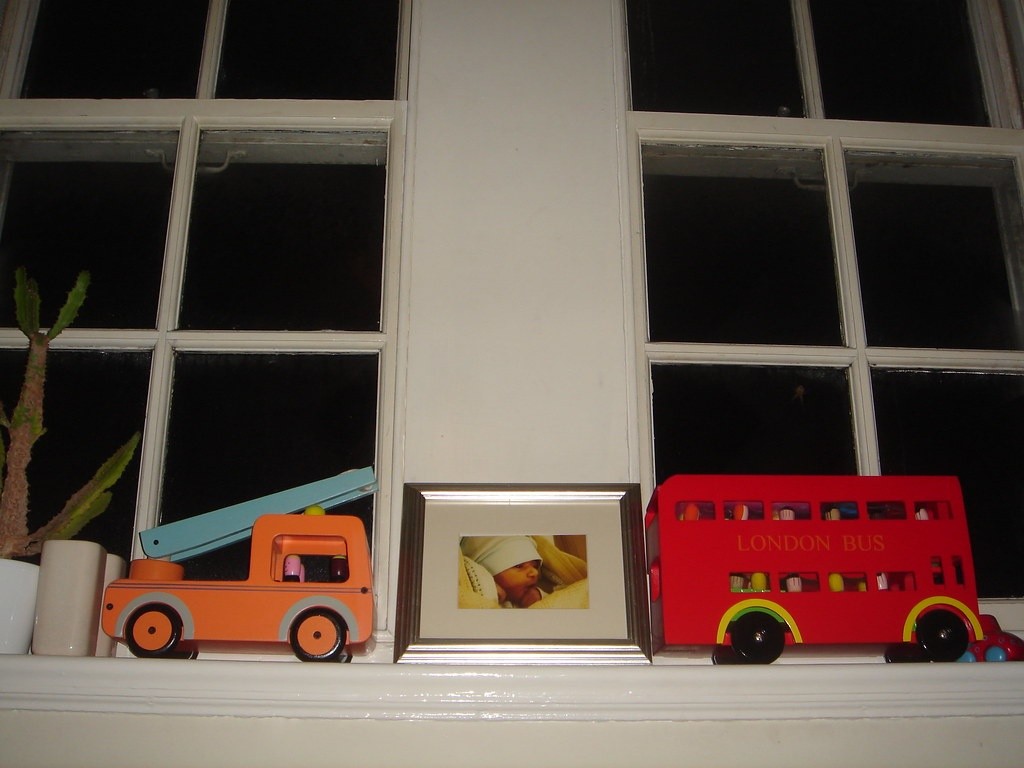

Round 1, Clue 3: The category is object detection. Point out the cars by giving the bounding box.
[884,613,1024,663]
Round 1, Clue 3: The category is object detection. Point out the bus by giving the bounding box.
[646,474,984,666]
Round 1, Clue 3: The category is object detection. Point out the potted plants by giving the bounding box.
[0,267,141,654]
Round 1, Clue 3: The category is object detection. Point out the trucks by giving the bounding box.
[101,465,373,663]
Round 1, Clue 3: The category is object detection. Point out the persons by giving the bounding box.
[459,536,548,609]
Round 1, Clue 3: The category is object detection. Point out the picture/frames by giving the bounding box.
[392,482,653,668]
